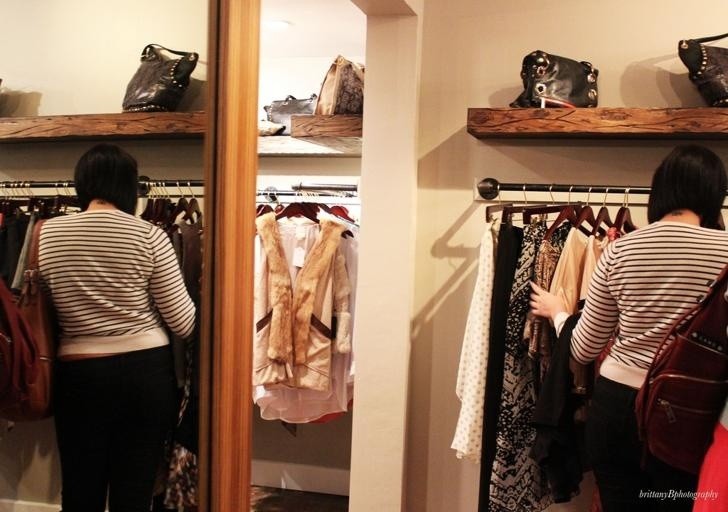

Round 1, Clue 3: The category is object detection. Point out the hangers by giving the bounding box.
[256,189,354,240]
[2,180,202,233]
[482,185,637,235]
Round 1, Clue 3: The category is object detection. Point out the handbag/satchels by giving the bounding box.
[679,34,728,108]
[122,43,200,114]
[510,49,599,111]
[317,55,365,114]
[263,91,321,135]
[632,263,728,477]
[0,216,60,420]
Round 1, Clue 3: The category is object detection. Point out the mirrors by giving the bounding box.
[0,0,259,510]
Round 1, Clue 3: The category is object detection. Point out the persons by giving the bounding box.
[528,147,728,512]
[38,144,196,512]
